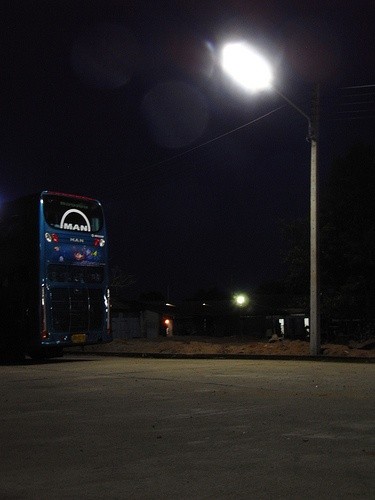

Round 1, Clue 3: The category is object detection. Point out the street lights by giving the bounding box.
[218,41,323,356]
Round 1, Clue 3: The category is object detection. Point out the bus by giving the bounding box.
[1,188,111,364]
